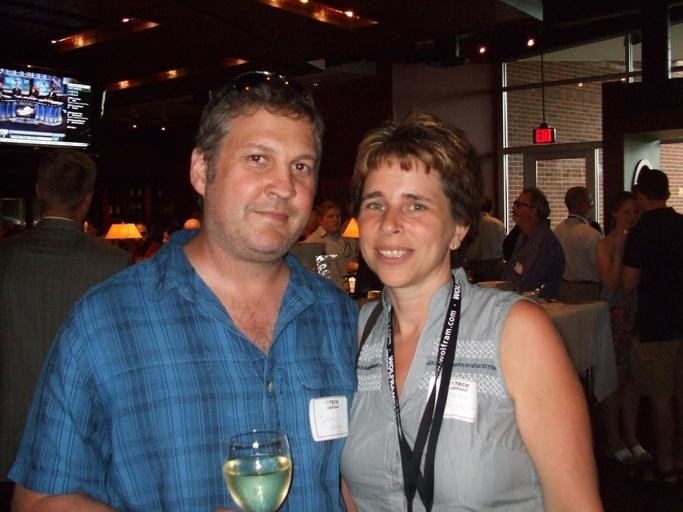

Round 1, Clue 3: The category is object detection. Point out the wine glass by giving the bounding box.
[222,431,293,510]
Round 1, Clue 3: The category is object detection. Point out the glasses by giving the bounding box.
[513,200,535,209]
[588,201,595,205]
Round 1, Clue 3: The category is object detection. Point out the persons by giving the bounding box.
[129,222,158,262]
[552,187,606,303]
[185,217,199,229]
[78,208,109,236]
[7,72,361,512]
[459,191,505,283]
[161,224,180,251]
[621,168,683,491]
[301,198,357,299]
[341,109,603,512]
[503,189,566,313]
[0,148,135,479]
[605,191,656,482]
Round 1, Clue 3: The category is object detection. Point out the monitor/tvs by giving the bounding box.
[0,65,97,150]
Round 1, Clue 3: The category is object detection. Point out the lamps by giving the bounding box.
[105,224,141,253]
[343,218,360,261]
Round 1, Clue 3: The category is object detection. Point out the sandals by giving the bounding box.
[630,445,647,457]
[608,444,633,476]
[625,459,681,491]
